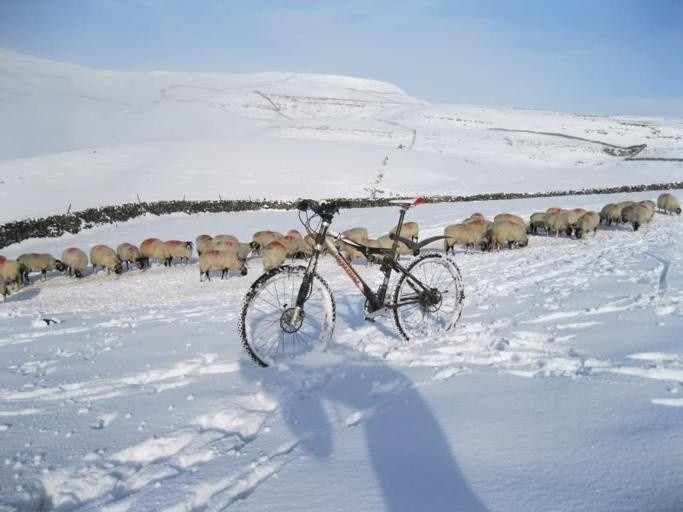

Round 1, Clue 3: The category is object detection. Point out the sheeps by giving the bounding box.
[139,237,173,267]
[0,255,22,303]
[116,242,146,271]
[16,252,65,282]
[89,244,122,275]
[658,191,683,216]
[196,222,421,283]
[442,200,656,256]
[164,240,193,265]
[60,247,89,277]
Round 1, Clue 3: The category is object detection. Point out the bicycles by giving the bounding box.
[239,196,465,369]
